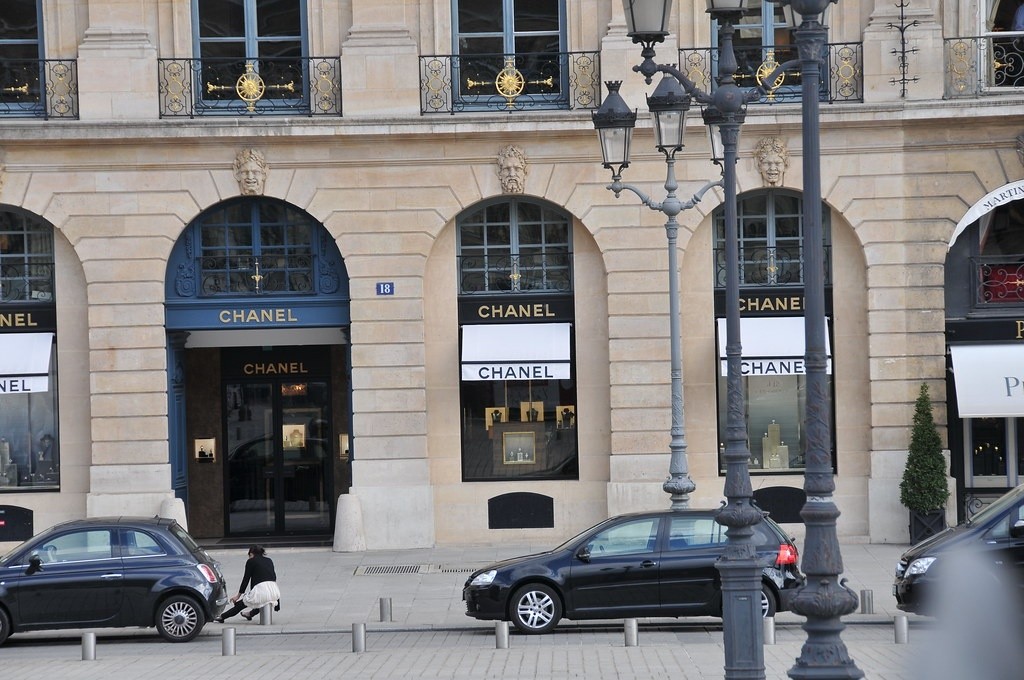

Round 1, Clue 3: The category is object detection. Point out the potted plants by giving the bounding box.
[900,381,952,545]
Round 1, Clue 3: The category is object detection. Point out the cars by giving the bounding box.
[461,507,808,634]
[891,484,1024,616]
[0,515,228,647]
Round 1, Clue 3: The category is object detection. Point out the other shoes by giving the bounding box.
[215,615,224,623]
[241,611,252,621]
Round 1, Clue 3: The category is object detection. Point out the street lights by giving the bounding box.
[592,66,739,511]
[620,1,864,680]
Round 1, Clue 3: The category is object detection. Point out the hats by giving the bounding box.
[40,434,55,441]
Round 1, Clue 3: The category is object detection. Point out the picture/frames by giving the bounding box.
[503,431,537,464]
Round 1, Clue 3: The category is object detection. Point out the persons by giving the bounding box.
[497,145,529,194]
[754,135,791,188]
[214,545,280,623]
[233,149,269,195]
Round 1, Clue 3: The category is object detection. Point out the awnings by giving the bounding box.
[460,322,570,381]
[948,345,1024,418]
[716,317,832,376]
[0,332,56,393]
